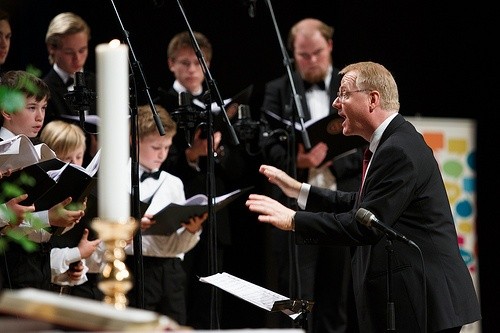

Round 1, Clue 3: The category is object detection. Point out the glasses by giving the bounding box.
[337,89,374,100]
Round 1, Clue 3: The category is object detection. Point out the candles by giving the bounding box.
[95,39,130,225]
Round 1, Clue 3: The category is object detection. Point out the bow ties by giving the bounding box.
[66,77,75,89]
[141,170,161,182]
[182,90,207,104]
[303,78,327,91]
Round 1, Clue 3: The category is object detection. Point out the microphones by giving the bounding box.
[355,208,411,246]
[173,92,199,146]
[63,71,96,116]
[235,104,262,145]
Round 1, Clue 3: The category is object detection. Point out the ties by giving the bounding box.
[361,147,373,190]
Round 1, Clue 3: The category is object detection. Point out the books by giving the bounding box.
[1,132,101,214]
[143,186,252,236]
[60,111,99,131]
[260,103,359,169]
[168,96,232,137]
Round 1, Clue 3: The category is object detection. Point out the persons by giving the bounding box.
[0,70,88,288]
[125,102,210,326]
[38,11,99,180]
[0,15,12,73]
[0,193,36,235]
[157,30,240,328]
[35,119,106,302]
[245,60,482,332]
[256,17,372,332]
